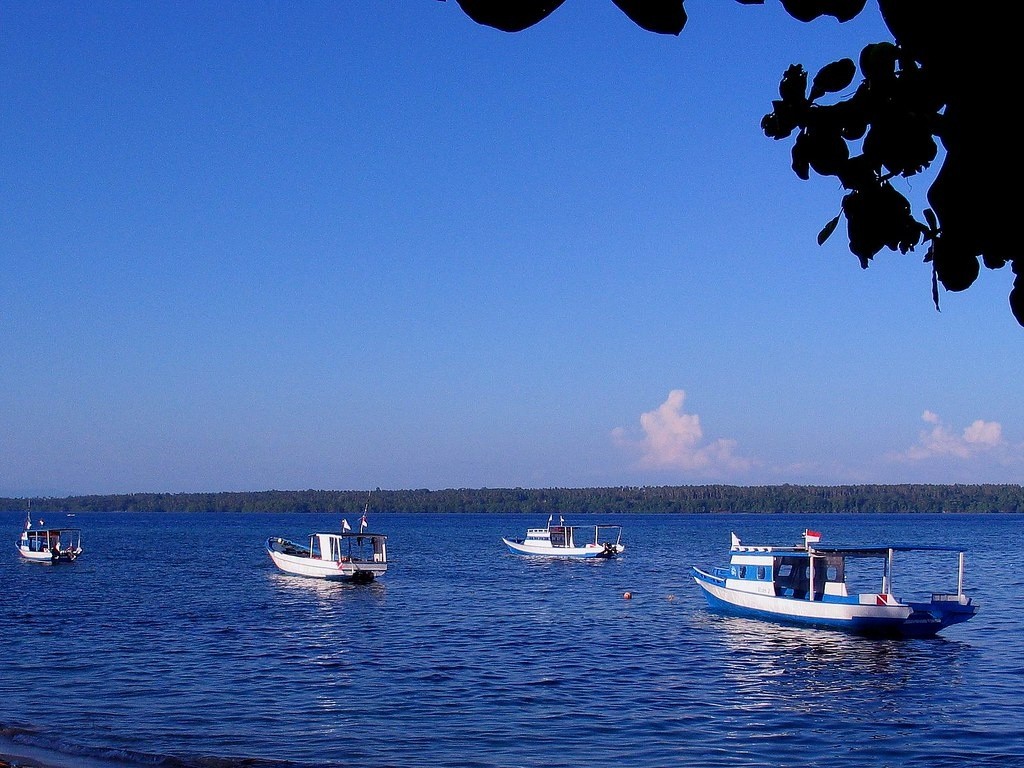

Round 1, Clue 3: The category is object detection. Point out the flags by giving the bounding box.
[21,532,28,540]
[806,530,822,543]
[24,521,32,530]
[362,515,368,527]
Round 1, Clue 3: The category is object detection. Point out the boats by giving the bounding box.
[691,544,980,639]
[264,532,389,582]
[502,525,625,560]
[15,528,83,565]
